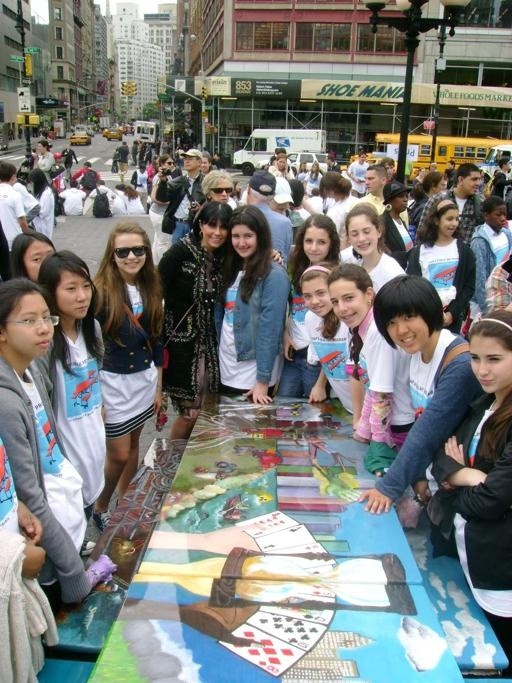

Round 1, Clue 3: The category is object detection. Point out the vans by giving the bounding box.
[133,120,159,145]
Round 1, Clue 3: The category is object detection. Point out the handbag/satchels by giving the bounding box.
[47,162,66,180]
[157,340,170,369]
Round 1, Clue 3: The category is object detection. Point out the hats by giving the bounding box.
[180,149,202,158]
[249,170,276,195]
[382,180,412,206]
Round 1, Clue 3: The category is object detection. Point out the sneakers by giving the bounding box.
[79,540,97,556]
[92,510,111,533]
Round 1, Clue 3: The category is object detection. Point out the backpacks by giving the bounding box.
[93,188,112,218]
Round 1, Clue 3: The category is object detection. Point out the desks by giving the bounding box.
[32,392,512,682]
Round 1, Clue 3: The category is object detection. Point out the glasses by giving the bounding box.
[163,162,174,165]
[209,187,232,193]
[0,315,60,327]
[114,246,148,258]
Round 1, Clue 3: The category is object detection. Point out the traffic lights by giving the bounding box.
[119,81,129,96]
[129,80,138,96]
[201,85,208,100]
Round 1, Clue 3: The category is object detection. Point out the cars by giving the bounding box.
[69,128,92,146]
[98,122,135,142]
[338,152,423,184]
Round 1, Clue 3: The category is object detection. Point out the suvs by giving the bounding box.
[261,148,333,176]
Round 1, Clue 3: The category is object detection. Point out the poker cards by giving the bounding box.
[217,510,335,677]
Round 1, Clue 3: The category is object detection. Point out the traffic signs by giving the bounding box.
[22,45,40,53]
[8,54,24,62]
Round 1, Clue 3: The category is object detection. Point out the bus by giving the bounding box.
[374,133,512,187]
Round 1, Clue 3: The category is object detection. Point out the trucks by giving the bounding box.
[474,141,512,182]
[232,128,327,176]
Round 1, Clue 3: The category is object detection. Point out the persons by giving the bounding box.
[1,128,512,683]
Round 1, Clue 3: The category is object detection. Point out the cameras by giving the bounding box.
[162,167,172,177]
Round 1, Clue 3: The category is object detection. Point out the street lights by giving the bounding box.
[429,22,450,165]
[188,33,209,152]
[14,8,35,165]
[360,0,472,193]
[76,75,92,122]
